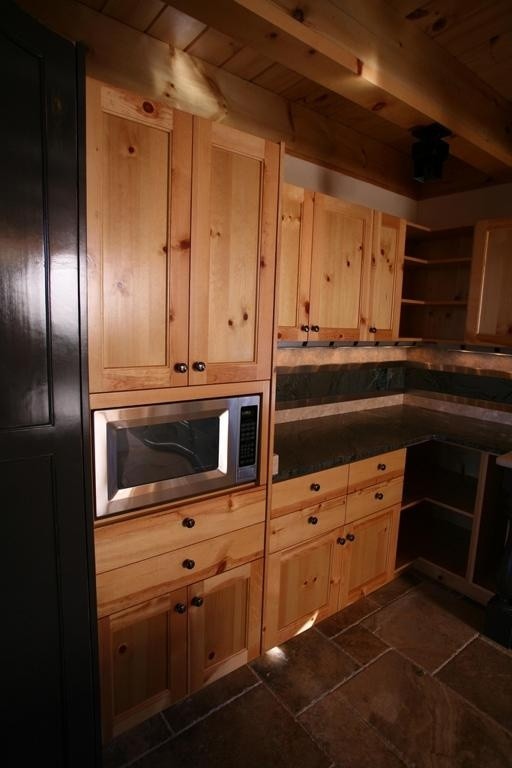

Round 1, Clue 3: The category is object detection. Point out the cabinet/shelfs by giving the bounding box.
[277,181,374,341]
[92,558,264,745]
[367,209,471,341]
[86,76,278,395]
[465,219,511,347]
[92,490,267,621]
[267,448,406,656]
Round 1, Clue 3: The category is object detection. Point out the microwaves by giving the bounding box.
[93,396,261,518]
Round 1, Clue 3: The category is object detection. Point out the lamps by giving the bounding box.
[407,121,451,182]
